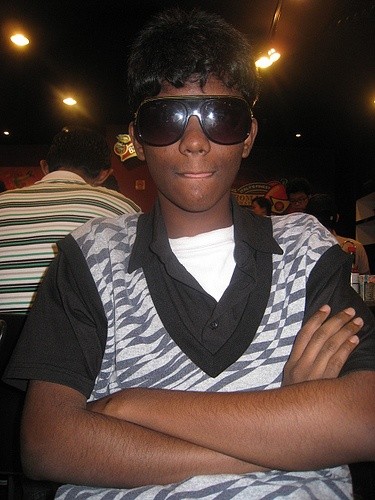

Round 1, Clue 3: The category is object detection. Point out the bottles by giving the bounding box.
[347,247,360,294]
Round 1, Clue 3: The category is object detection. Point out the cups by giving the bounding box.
[358,274,375,306]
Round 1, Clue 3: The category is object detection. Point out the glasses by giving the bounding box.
[289,196,308,206]
[132,94,253,146]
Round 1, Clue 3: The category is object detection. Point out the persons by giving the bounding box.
[251,197,273,218]
[285,178,310,213]
[0,129,145,500]
[2,11,375,500]
[304,195,371,275]
